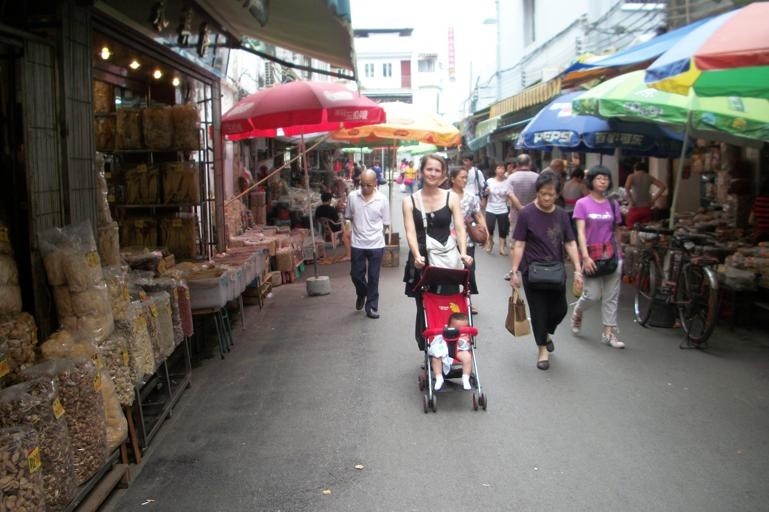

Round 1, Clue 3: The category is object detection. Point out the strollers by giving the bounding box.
[418,258,486,413]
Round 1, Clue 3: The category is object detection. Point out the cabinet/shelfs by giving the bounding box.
[91,112,207,258]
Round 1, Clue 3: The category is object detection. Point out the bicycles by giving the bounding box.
[634,228,720,350]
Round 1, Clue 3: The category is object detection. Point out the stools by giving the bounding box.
[188,308,233,359]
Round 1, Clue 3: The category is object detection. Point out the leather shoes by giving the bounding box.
[537,339,554,370]
[356,295,364,310]
[368,308,379,318]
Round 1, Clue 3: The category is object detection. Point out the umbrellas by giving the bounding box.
[570,69,769,227]
[642,0,768,103]
[513,88,698,165]
[305,99,461,244]
[209,78,388,279]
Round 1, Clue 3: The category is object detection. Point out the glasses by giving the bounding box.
[361,184,373,187]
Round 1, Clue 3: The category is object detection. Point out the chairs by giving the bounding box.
[320,217,344,249]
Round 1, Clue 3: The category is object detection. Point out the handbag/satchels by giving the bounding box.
[396,176,402,184]
[505,297,530,337]
[425,235,464,270]
[404,178,413,185]
[466,215,487,242]
[528,261,565,288]
[579,240,618,276]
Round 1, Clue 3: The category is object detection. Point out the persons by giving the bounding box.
[344,158,381,191]
[448,153,626,370]
[340,229,351,262]
[401,154,474,370]
[626,161,666,231]
[315,191,344,245]
[427,312,473,391]
[344,168,391,318]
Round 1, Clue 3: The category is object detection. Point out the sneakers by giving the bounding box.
[472,306,478,314]
[479,242,514,255]
[570,306,582,333]
[602,333,625,348]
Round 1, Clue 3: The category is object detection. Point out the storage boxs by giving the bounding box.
[187,247,263,310]
